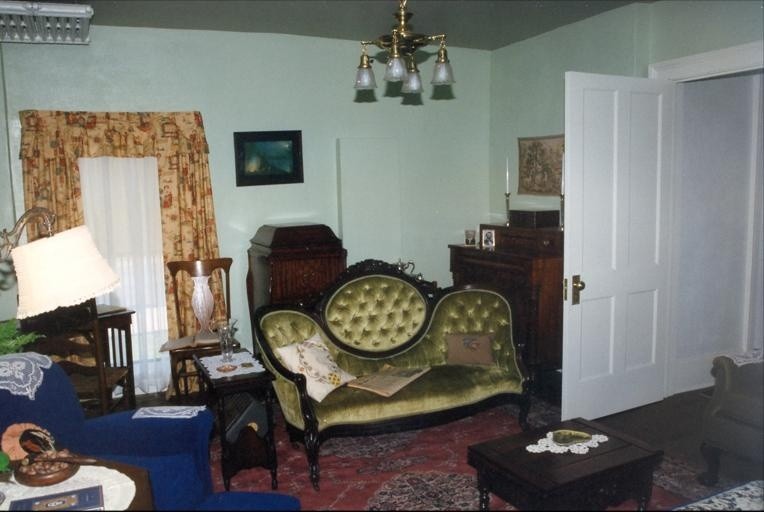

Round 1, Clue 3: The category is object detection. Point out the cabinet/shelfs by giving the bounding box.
[246,223,347,405]
[448,224,564,395]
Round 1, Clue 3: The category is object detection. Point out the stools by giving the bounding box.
[196,491,300,510]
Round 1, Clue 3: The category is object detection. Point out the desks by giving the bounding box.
[0,455,154,511]
[97,304,135,410]
[193,348,278,491]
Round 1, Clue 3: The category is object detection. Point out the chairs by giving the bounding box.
[18,298,128,416]
[0,352,212,511]
[696,356,764,486]
[167,258,232,405]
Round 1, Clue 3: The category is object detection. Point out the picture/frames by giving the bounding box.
[463,229,495,250]
[234,131,303,186]
[518,134,565,196]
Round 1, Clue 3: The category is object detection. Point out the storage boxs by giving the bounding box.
[510,211,559,229]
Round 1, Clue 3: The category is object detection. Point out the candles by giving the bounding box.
[561,153,564,195]
[506,158,509,194]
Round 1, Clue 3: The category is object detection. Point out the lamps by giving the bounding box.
[0,207,120,319]
[353,0,456,93]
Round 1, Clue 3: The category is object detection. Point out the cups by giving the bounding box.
[214,317,239,363]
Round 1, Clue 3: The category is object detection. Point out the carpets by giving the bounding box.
[210,395,746,510]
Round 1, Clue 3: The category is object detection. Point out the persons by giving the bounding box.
[484,232,493,245]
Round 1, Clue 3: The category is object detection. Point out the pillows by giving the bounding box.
[448,334,495,365]
[278,333,357,403]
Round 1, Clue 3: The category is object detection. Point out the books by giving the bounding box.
[8,484,104,511]
[347,364,431,399]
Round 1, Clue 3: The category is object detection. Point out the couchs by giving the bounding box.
[253,260,532,491]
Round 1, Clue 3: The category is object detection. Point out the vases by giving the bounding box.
[191,276,218,339]
